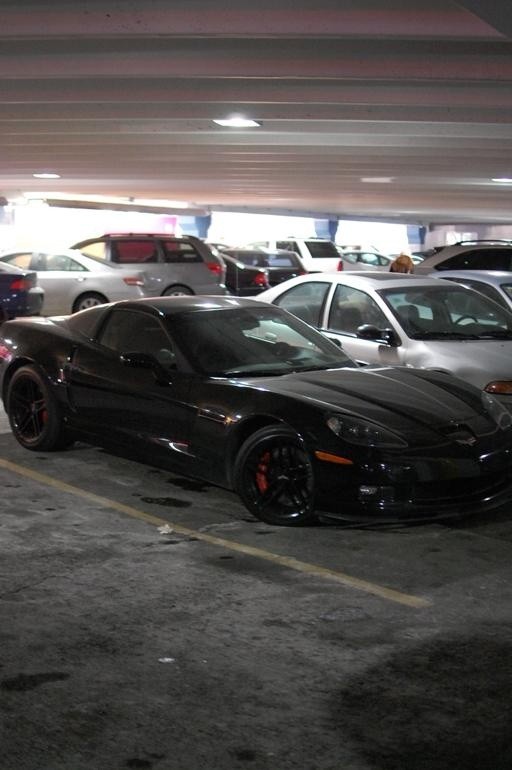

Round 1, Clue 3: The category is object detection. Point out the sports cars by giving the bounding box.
[0,293,512,532]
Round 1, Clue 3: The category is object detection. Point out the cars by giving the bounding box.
[245,269,511,410]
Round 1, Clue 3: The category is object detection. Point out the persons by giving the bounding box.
[390,255,415,274]
